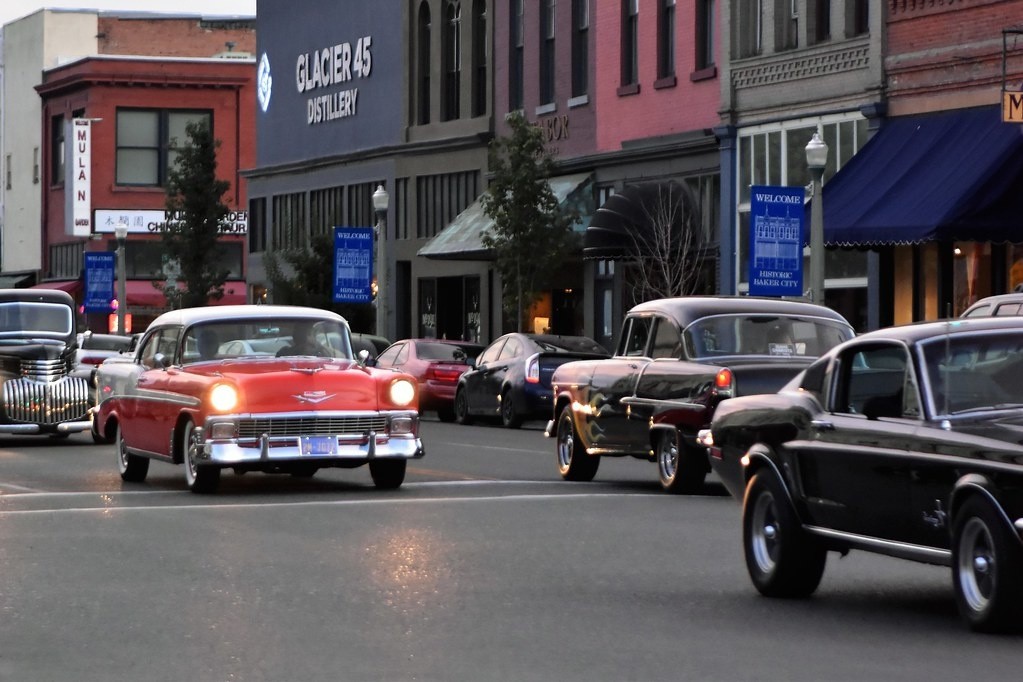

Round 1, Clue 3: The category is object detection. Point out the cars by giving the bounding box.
[453,331,613,428]
[375,339,489,422]
[92,304,426,494]
[549,303,871,495]
[0,289,394,438]
[705,292,1022,635]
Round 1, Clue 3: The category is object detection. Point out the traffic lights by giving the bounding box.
[77,297,85,316]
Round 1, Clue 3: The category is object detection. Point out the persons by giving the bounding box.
[768,325,797,355]
[275,321,346,358]
[198,331,220,361]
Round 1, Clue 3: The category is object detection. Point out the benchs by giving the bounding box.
[144,355,237,369]
[849,369,1004,421]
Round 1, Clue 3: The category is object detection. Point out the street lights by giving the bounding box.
[372,185,389,340]
[115,220,128,337]
[804,134,829,308]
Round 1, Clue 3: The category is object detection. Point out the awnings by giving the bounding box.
[113,280,249,311]
[0,275,80,298]
[417,171,599,263]
[804,103,1023,247]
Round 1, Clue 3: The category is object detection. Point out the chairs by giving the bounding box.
[764,329,794,356]
